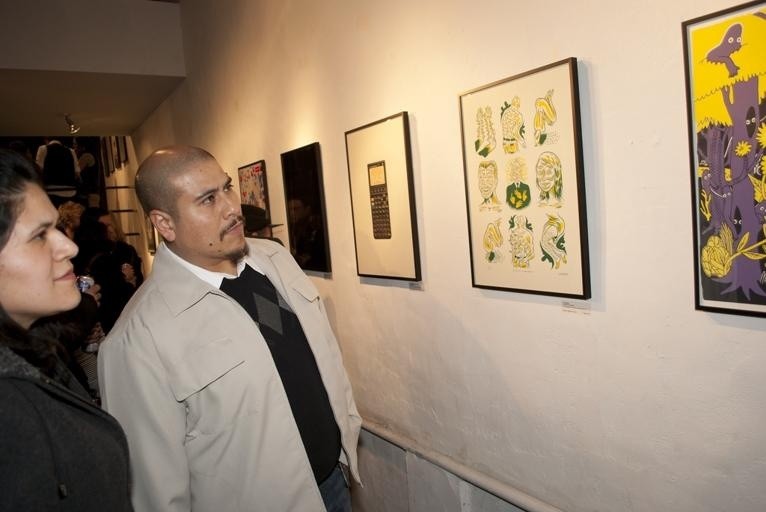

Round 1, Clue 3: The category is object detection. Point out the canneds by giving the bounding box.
[77,276,95,293]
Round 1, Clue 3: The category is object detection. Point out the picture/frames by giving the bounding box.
[460,57,592,301]
[344,112,421,280]
[279,142,332,278]
[680,1,765,318]
[238,160,274,240]
[101,135,129,178]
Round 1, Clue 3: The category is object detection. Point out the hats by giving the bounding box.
[241,204,283,231]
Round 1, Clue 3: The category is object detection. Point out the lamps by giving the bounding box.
[65,116,81,134]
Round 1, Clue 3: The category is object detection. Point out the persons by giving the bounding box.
[49,199,143,411]
[287,196,327,272]
[94,144,366,512]
[0,150,135,511]
[239,204,286,247]
